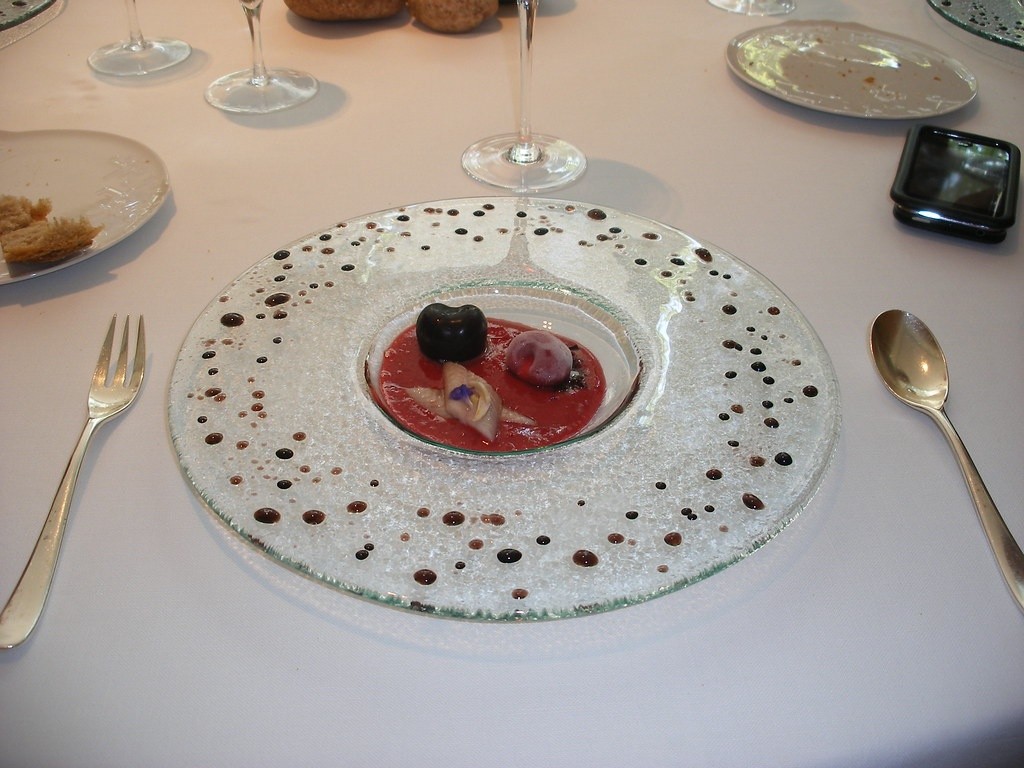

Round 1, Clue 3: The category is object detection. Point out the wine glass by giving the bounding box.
[459,0,588,192]
[205,0,320,112]
[88,0,192,76]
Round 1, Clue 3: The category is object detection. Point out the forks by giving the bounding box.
[0,312,147,646]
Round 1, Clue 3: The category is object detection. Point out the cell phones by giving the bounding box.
[889,123,1020,243]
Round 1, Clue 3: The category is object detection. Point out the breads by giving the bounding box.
[0,193,104,265]
[283,0,500,33]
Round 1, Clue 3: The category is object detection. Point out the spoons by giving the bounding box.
[869,309,1023,612]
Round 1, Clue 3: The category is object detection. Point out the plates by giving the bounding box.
[726,19,978,120]
[930,0,1023,49]
[1,0,65,47]
[0,128,169,287]
[167,198,844,621]
